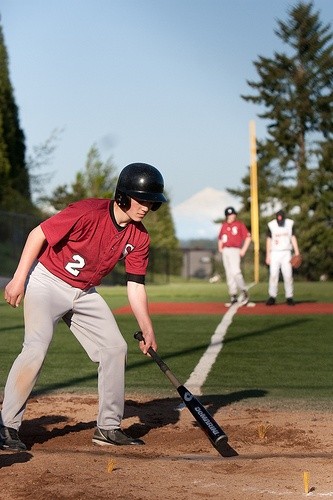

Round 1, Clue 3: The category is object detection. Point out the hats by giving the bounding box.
[225,207,237,215]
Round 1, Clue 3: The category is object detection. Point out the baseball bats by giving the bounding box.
[134,330,229,447]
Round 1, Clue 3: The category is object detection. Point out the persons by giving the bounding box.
[215,207,251,307]
[265,210,302,306]
[0,162,168,452]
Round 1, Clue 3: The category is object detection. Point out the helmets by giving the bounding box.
[114,162,167,213]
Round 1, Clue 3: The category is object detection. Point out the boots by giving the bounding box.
[239,290,250,305]
[224,295,238,307]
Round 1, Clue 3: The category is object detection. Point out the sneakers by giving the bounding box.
[0,411,27,452]
[91,428,146,447]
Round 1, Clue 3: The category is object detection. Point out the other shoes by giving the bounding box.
[266,297,276,305]
[287,298,295,305]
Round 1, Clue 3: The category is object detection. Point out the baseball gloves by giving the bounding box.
[290,256,302,268]
